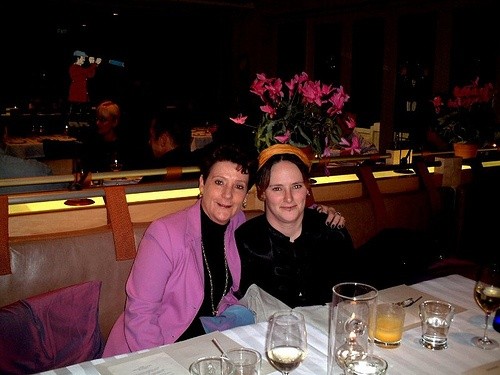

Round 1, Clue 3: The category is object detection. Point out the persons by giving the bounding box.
[102,142,345,359]
[231,143,358,313]
[0,50,500,195]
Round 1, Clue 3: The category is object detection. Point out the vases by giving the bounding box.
[301,147,313,167]
[454,142,479,160]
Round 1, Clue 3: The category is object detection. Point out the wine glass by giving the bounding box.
[470,264,500,351]
[265,310,308,375]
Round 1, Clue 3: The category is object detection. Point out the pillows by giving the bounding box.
[0,280,106,375]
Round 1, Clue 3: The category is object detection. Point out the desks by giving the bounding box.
[32,275,500,375]
[0,124,213,177]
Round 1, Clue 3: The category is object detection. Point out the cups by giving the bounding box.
[370,303,406,349]
[342,353,388,375]
[221,347,262,375]
[420,299,455,350]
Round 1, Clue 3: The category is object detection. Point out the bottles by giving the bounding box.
[189,356,236,375]
[327,281,378,375]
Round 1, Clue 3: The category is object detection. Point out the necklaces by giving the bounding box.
[202,229,229,315]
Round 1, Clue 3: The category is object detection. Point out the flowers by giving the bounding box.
[429,79,500,145]
[227,72,358,174]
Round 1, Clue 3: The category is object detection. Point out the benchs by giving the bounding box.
[0,177,500,340]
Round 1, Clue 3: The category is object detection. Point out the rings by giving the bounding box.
[337,211,341,216]
